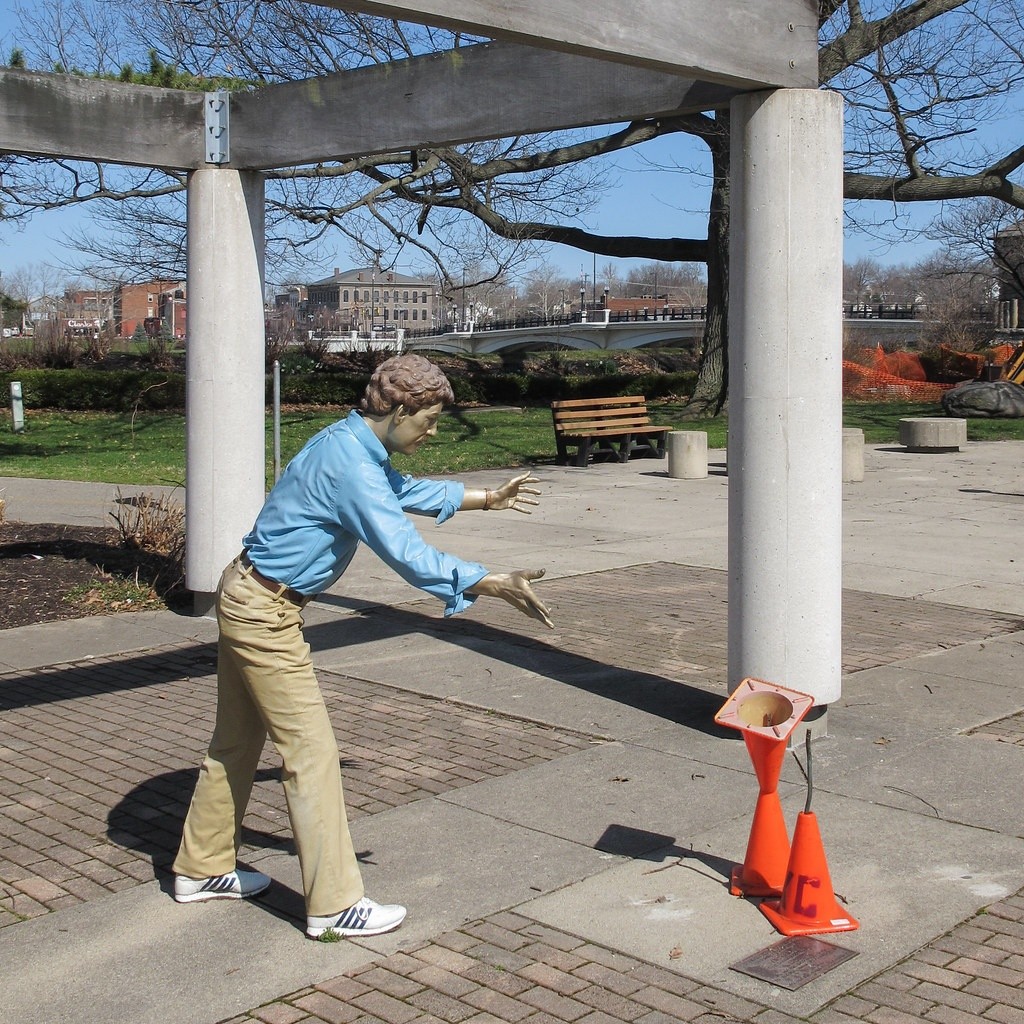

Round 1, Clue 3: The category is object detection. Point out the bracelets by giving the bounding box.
[483,488,491,510]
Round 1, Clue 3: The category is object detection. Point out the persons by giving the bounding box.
[171,353,556,937]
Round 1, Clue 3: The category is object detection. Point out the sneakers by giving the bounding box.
[175,869,271,903]
[306,894,407,939]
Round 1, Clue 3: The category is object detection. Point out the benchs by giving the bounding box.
[550,396,673,467]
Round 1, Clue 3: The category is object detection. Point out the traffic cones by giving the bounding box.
[714,677,860,937]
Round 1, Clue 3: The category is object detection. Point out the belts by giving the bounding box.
[240,549,317,603]
